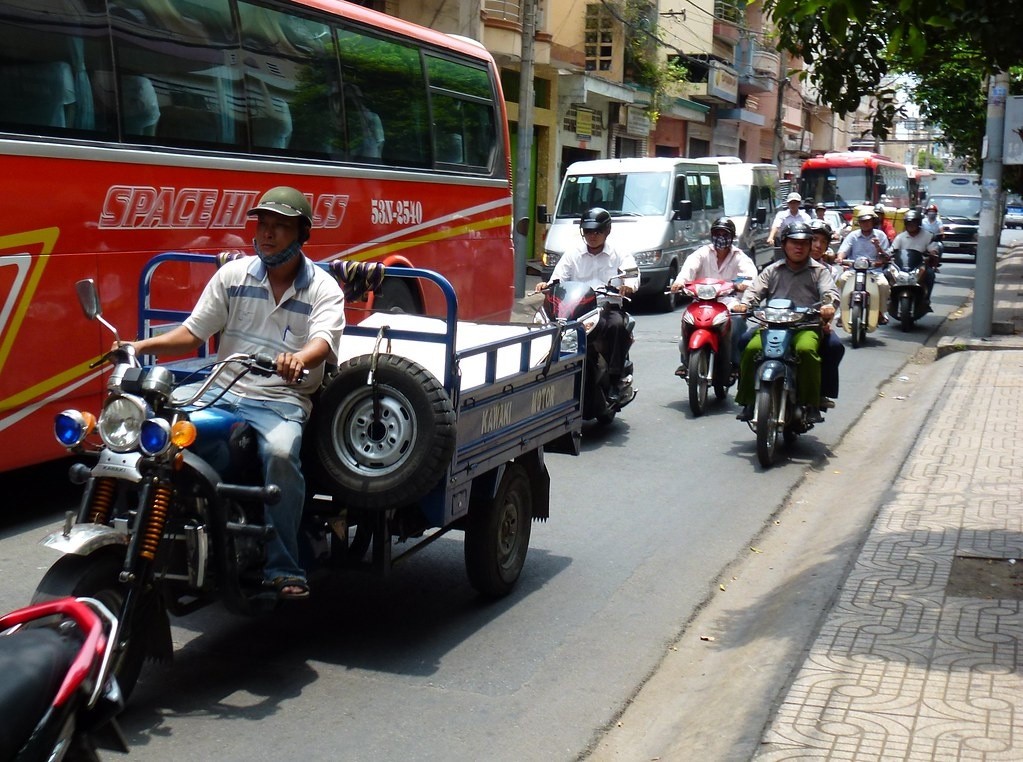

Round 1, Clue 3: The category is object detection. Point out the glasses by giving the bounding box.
[583,228,605,234]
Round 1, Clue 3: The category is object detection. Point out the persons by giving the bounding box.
[731,219,844,424]
[110,186,344,600]
[672,217,759,383]
[767,192,839,264]
[834,202,945,327]
[536,208,640,402]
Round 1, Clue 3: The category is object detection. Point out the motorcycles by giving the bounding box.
[834,256,892,348]
[54,249,590,699]
[725,298,836,469]
[662,253,755,417]
[877,248,941,334]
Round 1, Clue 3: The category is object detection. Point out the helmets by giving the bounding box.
[807,219,832,243]
[815,202,826,211]
[904,210,922,226]
[781,222,812,242]
[247,186,312,230]
[927,205,937,212]
[711,217,735,236]
[581,207,611,229]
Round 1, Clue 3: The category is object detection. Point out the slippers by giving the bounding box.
[275,576,309,600]
[675,365,687,376]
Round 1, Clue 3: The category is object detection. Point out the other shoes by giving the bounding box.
[607,387,620,402]
[836,319,842,327]
[879,314,889,324]
[736,404,754,420]
[819,396,835,408]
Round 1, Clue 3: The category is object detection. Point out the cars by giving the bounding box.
[803,207,852,253]
[911,193,1002,263]
[1002,204,1023,229]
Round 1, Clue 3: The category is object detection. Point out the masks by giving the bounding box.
[712,236,732,250]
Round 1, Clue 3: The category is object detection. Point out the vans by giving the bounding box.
[537,155,727,310]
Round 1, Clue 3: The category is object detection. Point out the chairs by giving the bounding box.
[32,61,462,166]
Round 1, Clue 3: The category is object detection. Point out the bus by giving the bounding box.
[0,0,516,475]
[903,164,937,211]
[692,157,779,255]
[796,150,927,240]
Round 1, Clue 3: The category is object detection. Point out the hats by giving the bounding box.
[857,209,877,220]
[804,197,814,206]
[874,206,883,213]
[787,192,802,203]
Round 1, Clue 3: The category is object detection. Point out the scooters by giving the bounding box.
[0,594,131,762]
[523,260,640,436]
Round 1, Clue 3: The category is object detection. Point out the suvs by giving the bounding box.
[915,172,984,204]
